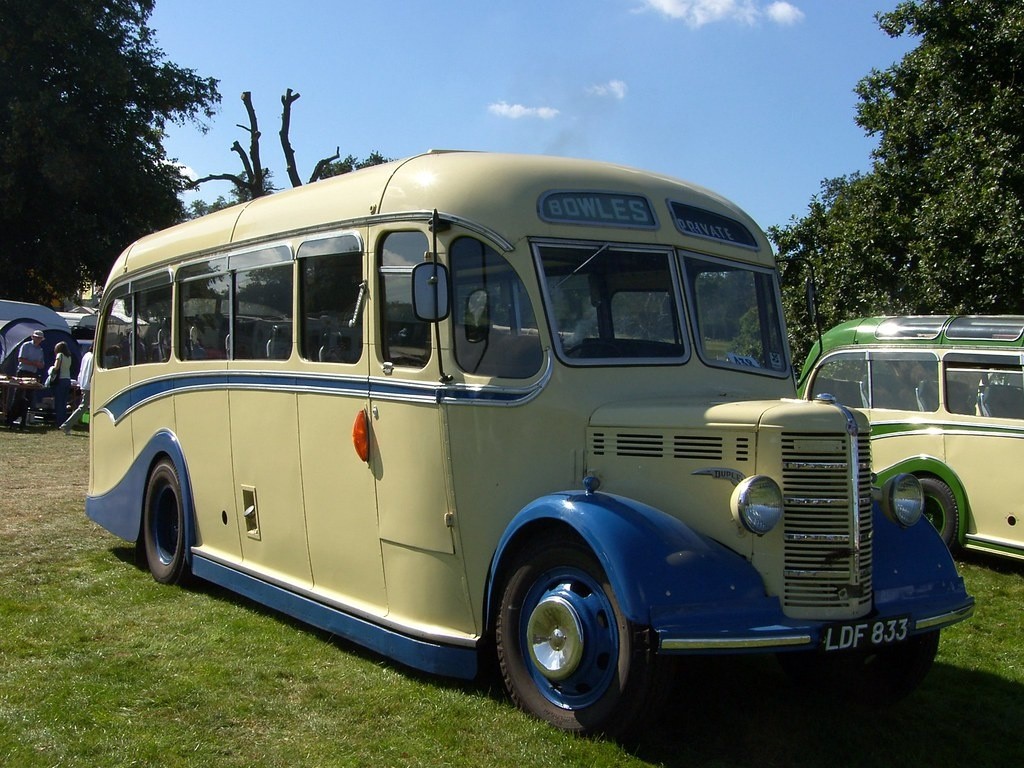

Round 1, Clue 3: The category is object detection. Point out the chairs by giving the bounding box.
[105,315,543,377]
[825,373,1024,420]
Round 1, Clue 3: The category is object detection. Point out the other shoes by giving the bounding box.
[60,424,72,434]
[7,423,14,430]
[17,424,30,432]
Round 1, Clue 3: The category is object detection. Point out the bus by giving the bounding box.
[81,145,976,735]
[795,312,1024,565]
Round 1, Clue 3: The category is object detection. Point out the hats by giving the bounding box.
[31,330,45,340]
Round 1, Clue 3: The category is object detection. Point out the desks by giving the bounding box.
[0,380,80,432]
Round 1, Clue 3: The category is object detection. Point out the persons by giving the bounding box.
[7,330,44,428]
[48,341,71,428]
[59,342,94,431]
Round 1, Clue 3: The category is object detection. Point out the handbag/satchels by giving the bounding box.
[50,368,59,386]
